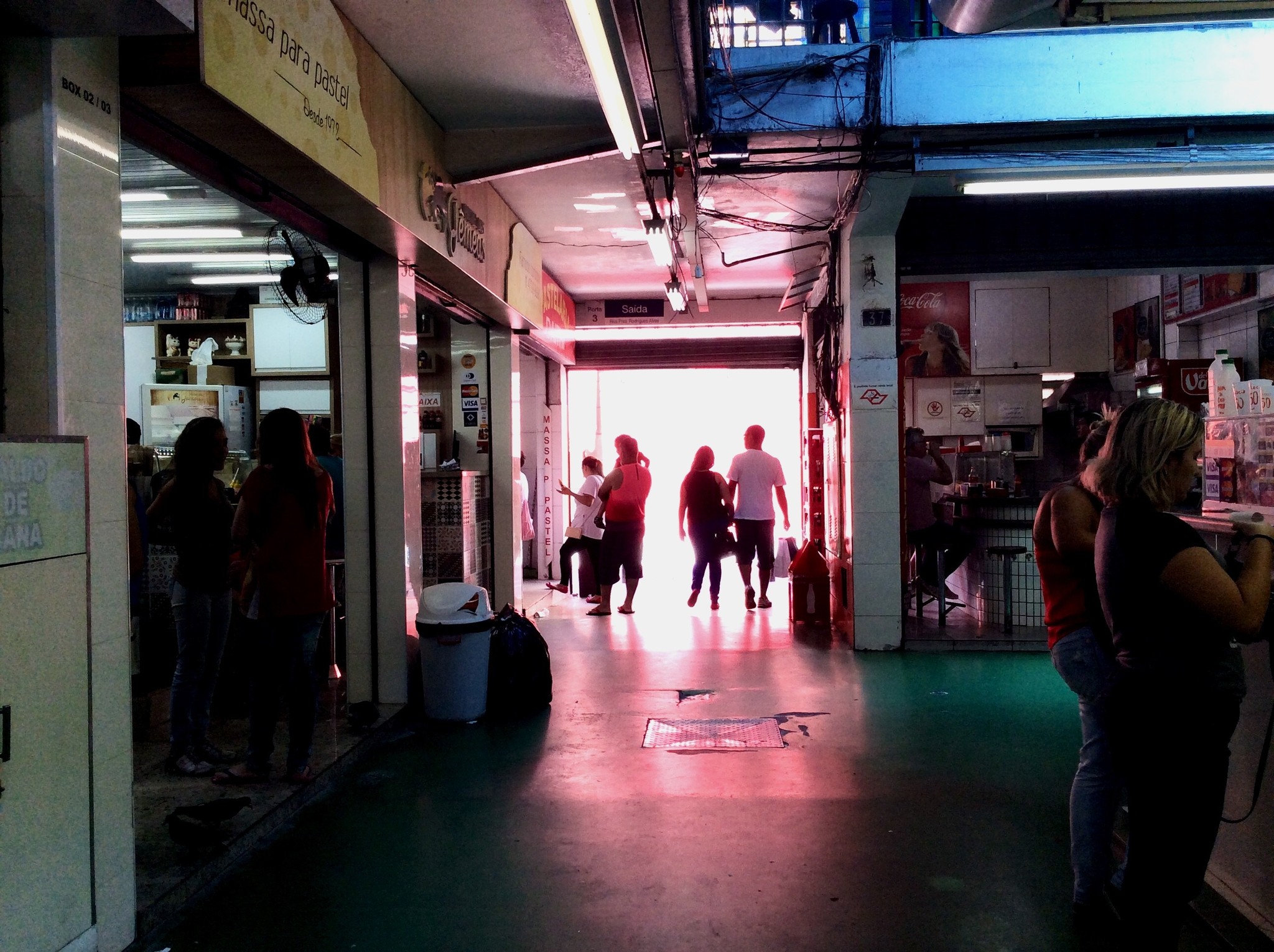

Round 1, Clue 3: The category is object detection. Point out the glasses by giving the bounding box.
[914,439,926,445]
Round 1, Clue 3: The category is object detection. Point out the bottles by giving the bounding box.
[1208,350,1229,417]
[985,432,1012,452]
[1217,359,1241,417]
[1014,477,1022,497]
[968,466,979,483]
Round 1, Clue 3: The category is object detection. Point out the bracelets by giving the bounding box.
[1246,534,1274,545]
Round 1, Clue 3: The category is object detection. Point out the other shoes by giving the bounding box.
[922,575,958,599]
[586,596,602,603]
[758,597,772,609]
[745,585,756,609]
[688,589,699,607]
[711,603,719,610]
[546,582,568,593]
[911,575,932,596]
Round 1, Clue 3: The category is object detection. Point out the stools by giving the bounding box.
[986,544,1027,638]
[907,534,966,626]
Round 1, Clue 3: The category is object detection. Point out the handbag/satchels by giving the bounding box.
[565,527,582,539]
[715,531,736,556]
[1222,541,1274,644]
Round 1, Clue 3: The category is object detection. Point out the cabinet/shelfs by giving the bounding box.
[153,319,342,459]
[417,333,446,465]
[969,275,1161,377]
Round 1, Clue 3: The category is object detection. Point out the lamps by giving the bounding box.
[955,159,1274,197]
[664,266,689,315]
[563,0,648,161]
[640,214,673,267]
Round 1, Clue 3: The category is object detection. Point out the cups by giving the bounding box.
[960,485,968,496]
[1248,379,1273,413]
[1231,381,1249,416]
[1259,385,1273,415]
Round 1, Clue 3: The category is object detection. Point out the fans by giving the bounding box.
[262,223,339,327]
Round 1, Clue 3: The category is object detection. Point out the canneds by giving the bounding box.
[175,293,205,320]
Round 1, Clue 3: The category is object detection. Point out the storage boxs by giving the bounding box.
[188,365,235,387]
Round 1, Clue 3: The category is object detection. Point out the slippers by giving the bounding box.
[619,607,633,614]
[210,767,272,785]
[283,766,315,785]
[586,608,611,616]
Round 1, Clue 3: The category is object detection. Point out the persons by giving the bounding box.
[546,433,652,617]
[1026,394,1274,952]
[904,426,982,600]
[678,445,735,610]
[900,321,970,377]
[520,451,536,541]
[125,407,342,786]
[724,424,790,609]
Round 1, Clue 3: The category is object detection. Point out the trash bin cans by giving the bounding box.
[787,538,831,626]
[415,581,493,726]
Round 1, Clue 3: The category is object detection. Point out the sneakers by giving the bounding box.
[195,739,225,763]
[594,517,606,529]
[163,746,216,775]
